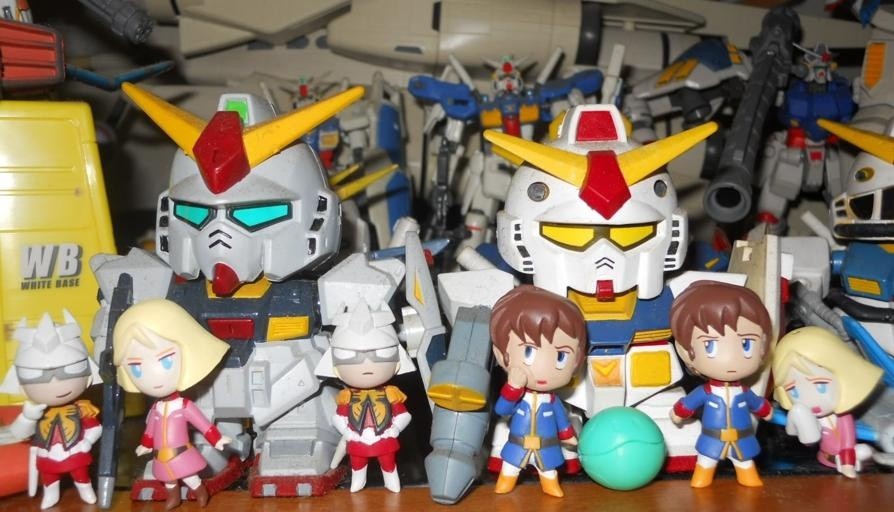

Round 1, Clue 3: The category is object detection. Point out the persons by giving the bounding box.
[0,0,894,512]
